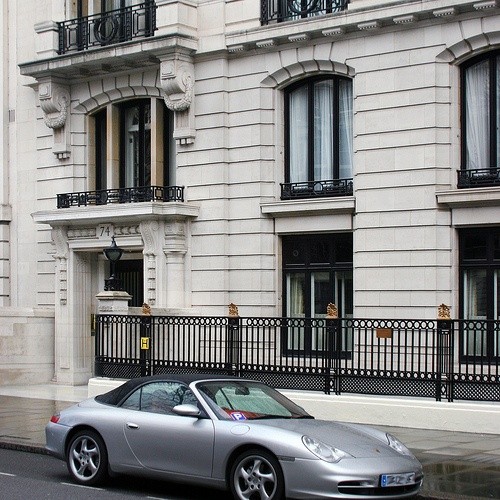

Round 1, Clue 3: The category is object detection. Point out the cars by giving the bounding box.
[44,375,426,500]
